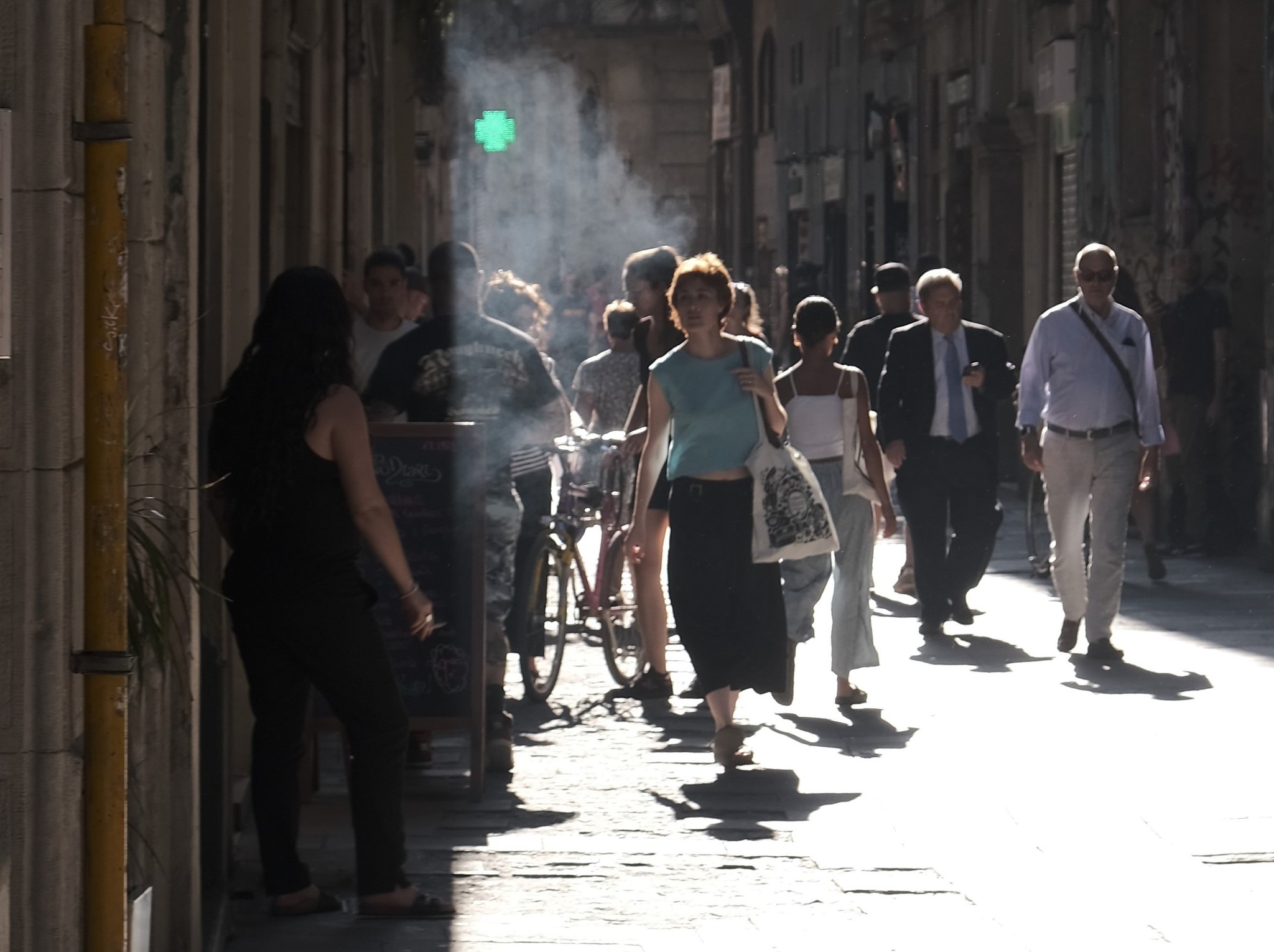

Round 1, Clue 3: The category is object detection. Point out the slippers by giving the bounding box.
[358,888,455,920]
[835,689,866,704]
[771,638,795,705]
[268,890,347,917]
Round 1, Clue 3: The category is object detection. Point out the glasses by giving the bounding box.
[1078,270,1113,283]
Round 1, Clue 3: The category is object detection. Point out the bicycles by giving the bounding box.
[514,436,648,701]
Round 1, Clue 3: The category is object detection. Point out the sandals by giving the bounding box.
[628,669,673,699]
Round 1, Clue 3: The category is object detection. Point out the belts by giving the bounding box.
[1047,420,1131,441]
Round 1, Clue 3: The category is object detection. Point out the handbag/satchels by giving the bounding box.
[743,335,840,563]
[842,366,885,507]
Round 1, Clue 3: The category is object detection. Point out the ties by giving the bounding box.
[943,336,967,445]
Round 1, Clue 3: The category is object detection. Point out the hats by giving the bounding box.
[870,263,911,295]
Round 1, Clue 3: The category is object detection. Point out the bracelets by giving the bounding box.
[400,584,419,599]
[1020,426,1037,439]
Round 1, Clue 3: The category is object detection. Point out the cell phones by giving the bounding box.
[963,362,980,376]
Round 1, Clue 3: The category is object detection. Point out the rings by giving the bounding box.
[749,379,752,385]
[750,373,753,378]
[426,614,432,622]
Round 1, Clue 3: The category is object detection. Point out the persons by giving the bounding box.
[624,255,788,766]
[207,267,455,916]
[549,246,1014,706]
[1014,243,1166,660]
[347,242,571,772]
[1114,248,1233,579]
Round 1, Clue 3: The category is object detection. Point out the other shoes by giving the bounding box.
[714,724,753,766]
[1057,618,1080,651]
[1088,639,1123,659]
[1145,551,1166,579]
[919,608,949,635]
[950,592,973,624]
[1170,544,1207,558]
[408,751,436,768]
[896,564,916,593]
[484,729,514,773]
